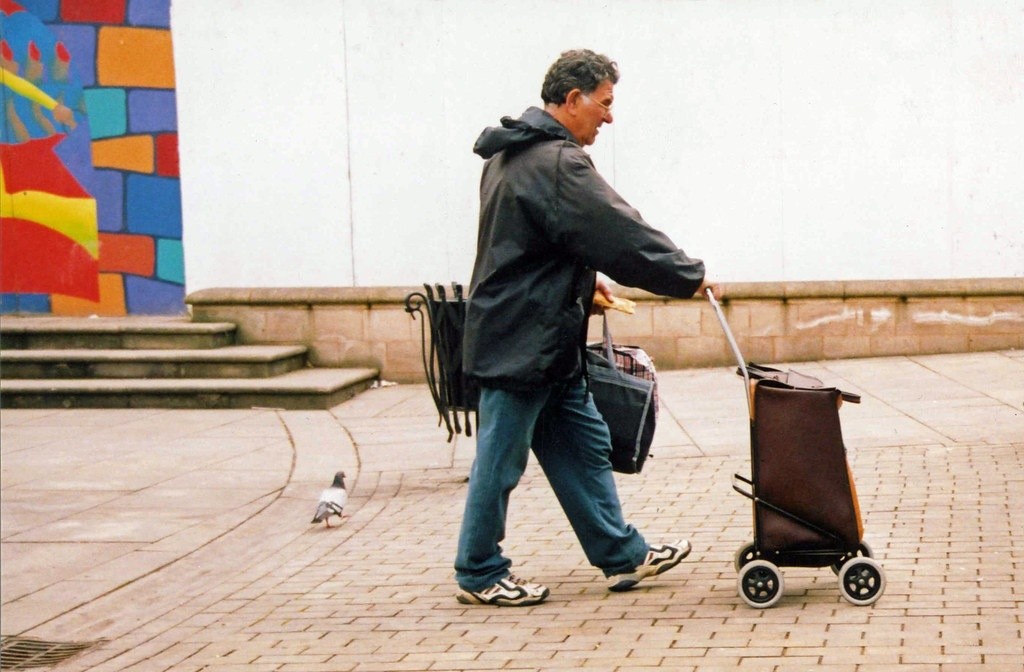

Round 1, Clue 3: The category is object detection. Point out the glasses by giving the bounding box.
[583,91,615,117]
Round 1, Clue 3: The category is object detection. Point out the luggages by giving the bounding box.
[706,288,886,606]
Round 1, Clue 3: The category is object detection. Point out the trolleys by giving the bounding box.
[706,288,886,609]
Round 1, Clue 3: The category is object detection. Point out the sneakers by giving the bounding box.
[608,540,690,591]
[457,574,549,606]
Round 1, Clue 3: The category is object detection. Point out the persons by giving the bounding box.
[453,49,711,604]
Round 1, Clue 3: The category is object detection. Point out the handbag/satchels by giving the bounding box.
[581,300,657,473]
[582,306,659,416]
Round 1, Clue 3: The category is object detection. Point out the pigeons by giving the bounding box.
[310,472,348,528]
